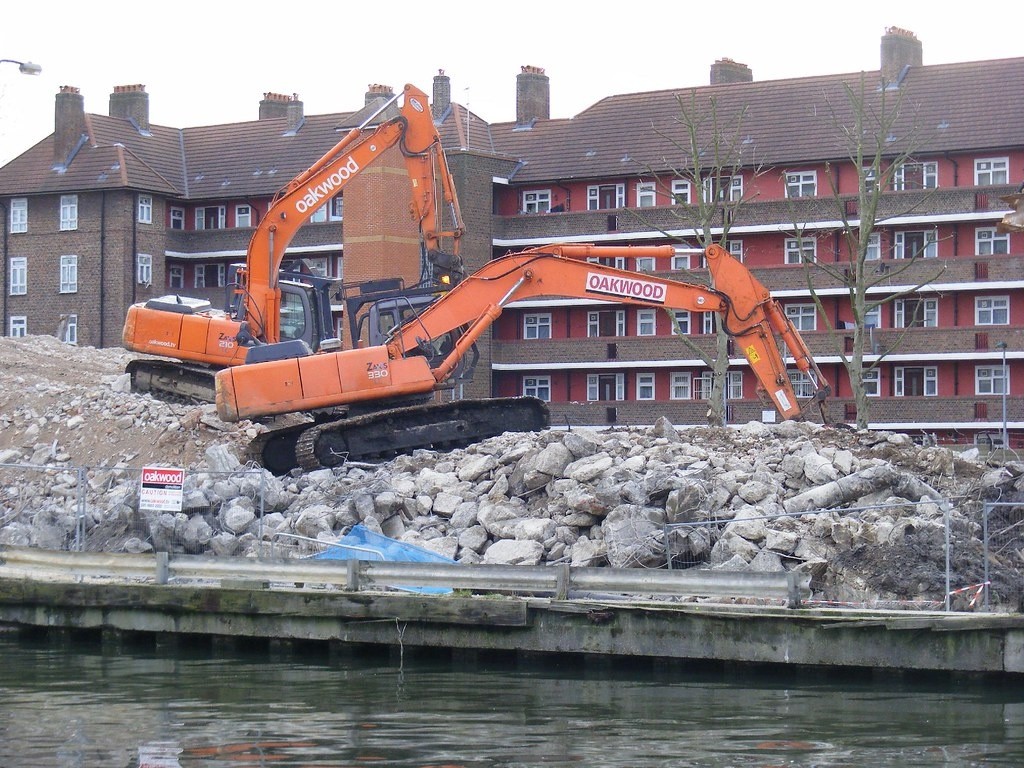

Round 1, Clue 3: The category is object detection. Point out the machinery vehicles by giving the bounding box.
[212,241,855,478]
[121,82,468,407]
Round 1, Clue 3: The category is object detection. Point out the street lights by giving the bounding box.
[993,342,1008,451]
